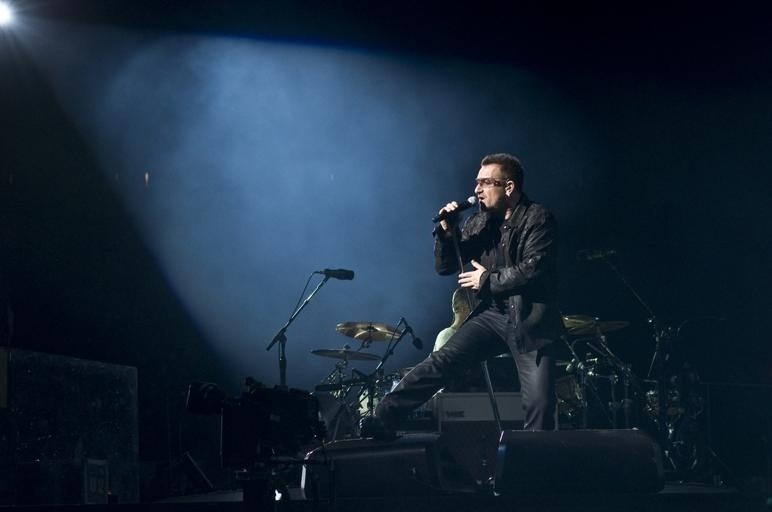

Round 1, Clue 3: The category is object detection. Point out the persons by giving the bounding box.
[375,154,560,429]
[433,287,483,395]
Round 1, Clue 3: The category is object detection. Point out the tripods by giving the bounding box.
[236,346,300,503]
[654,342,686,484]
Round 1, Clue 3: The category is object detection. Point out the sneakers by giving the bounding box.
[361,414,385,439]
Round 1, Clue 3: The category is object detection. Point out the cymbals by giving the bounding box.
[568,320,631,336]
[336,321,402,342]
[310,349,381,360]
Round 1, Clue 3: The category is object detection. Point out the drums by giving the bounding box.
[643,388,698,414]
[554,373,614,420]
[391,367,441,438]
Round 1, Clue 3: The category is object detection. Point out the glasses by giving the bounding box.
[474,178,504,187]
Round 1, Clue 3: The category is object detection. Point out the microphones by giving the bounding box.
[432,196,477,223]
[320,268,354,281]
[401,317,423,350]
[575,248,617,265]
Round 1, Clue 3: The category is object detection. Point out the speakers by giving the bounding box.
[0,347,142,507]
[707,384,772,488]
[494,430,664,494]
[300,432,444,497]
